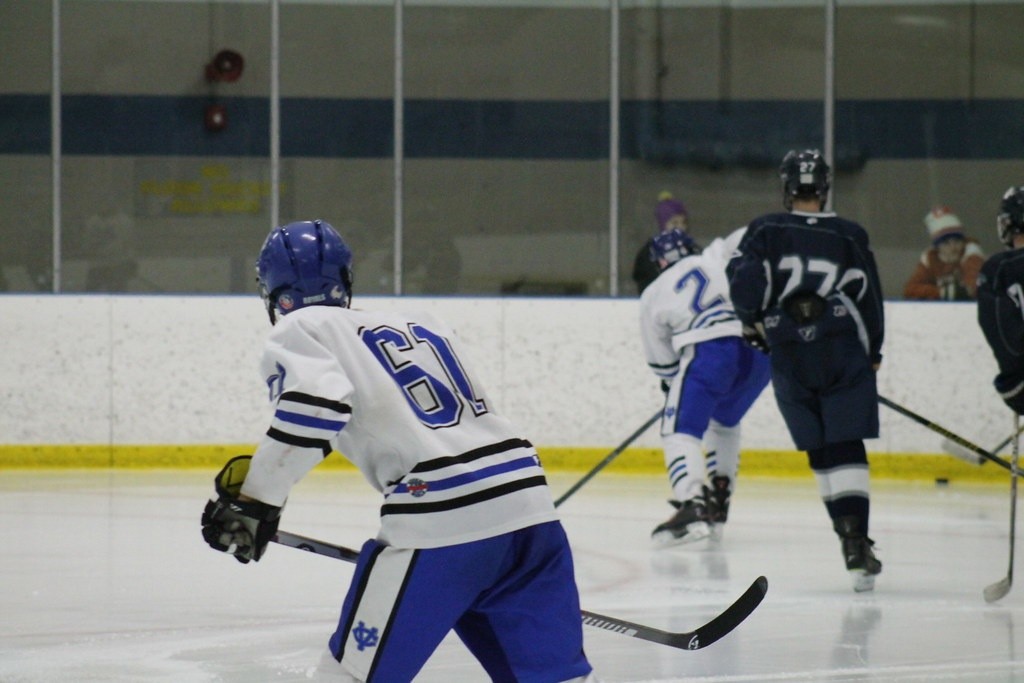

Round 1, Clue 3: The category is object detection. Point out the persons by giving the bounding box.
[201,220,592,683]
[632,190,700,293]
[638,225,772,539]
[903,208,990,301]
[725,149,884,574]
[976,186,1024,417]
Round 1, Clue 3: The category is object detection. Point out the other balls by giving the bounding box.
[936,479,948,483]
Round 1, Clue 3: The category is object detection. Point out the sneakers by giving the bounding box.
[651,485,712,551]
[710,475,732,541]
[836,517,881,591]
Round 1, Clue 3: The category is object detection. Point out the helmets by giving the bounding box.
[649,228,694,274]
[254,220,353,327]
[997,186,1024,247]
[777,148,830,210]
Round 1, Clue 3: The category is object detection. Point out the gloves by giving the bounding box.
[201,455,283,564]
[736,309,768,351]
[662,379,670,392]
[871,354,881,371]
[995,373,1024,415]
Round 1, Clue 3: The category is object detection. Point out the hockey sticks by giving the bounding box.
[983,412,1020,604]
[552,407,666,508]
[875,393,1024,479]
[225,518,772,652]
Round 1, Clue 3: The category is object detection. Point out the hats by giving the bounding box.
[923,207,964,240]
[655,191,689,234]
